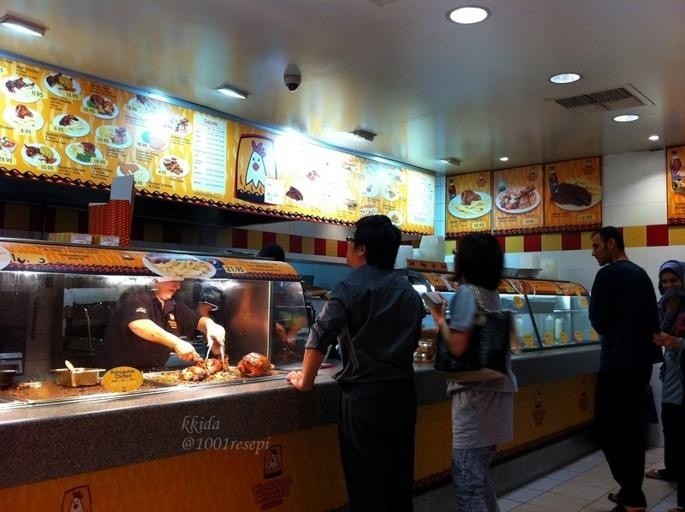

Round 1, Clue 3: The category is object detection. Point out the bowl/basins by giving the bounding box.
[395,234,455,273]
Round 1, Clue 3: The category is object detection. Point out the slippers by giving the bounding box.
[646,469,676,482]
[668,507,685,512]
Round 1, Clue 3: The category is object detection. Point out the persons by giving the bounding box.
[645,259,682,484]
[419,229,523,512]
[588,224,665,510]
[284,214,426,506]
[258,241,309,343]
[97,274,227,379]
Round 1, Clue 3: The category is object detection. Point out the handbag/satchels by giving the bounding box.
[433,311,512,381]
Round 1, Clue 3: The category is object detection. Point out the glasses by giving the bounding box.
[451,248,455,254]
[346,236,355,241]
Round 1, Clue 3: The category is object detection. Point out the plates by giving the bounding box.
[142,253,217,279]
[552,183,601,211]
[360,174,404,227]
[0,246,11,270]
[447,192,491,219]
[495,190,541,214]
[0,71,191,185]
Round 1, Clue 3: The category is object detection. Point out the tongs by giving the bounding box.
[204,338,226,371]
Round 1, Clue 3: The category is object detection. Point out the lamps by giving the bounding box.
[0,15,47,38]
[442,158,460,167]
[346,129,376,142]
[213,84,247,101]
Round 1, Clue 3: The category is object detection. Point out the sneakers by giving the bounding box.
[608,493,647,507]
[610,504,648,512]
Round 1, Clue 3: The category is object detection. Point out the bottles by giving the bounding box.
[543,313,554,339]
[670,150,682,192]
[514,314,524,339]
[412,339,436,364]
